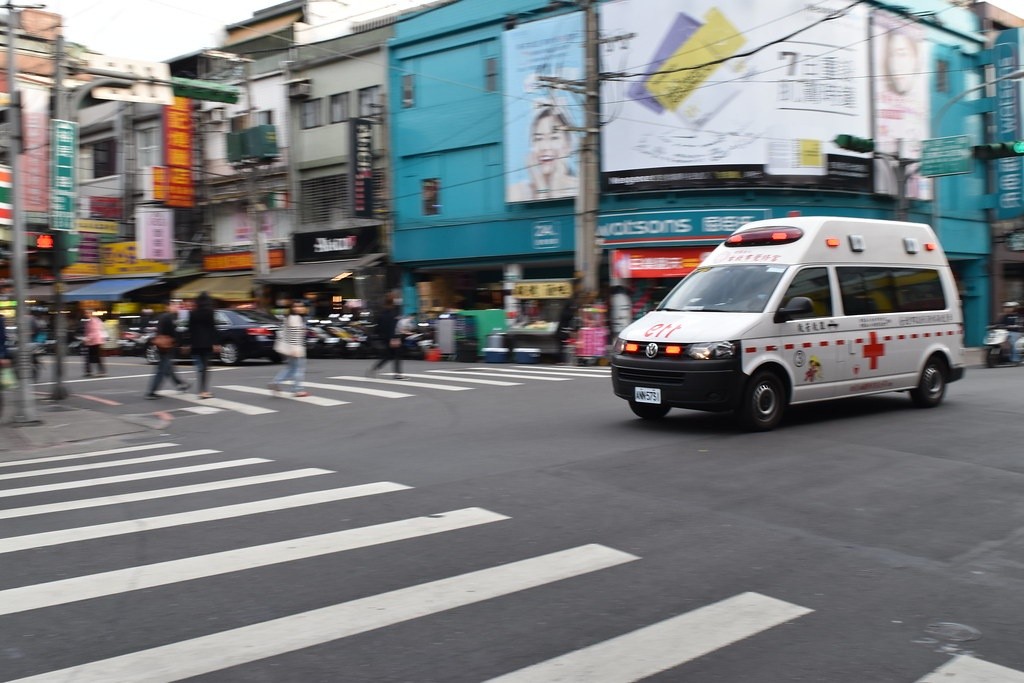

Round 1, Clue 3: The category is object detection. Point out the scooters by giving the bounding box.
[983,319,1024,367]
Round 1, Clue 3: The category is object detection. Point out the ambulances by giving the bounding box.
[607,215,970,433]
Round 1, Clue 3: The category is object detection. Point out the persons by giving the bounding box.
[508,108,577,202]
[76,310,110,377]
[394,313,416,335]
[267,299,311,396]
[0,314,11,417]
[144,301,192,399]
[366,295,411,381]
[187,293,222,398]
[996,301,1024,362]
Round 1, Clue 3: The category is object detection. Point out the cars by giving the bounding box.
[290,312,437,357]
[113,306,281,366]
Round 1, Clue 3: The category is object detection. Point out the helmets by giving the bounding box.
[1003,301,1019,306]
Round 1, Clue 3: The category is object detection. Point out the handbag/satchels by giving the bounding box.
[389,338,402,347]
[273,331,286,354]
[151,335,174,347]
[0,366,20,390]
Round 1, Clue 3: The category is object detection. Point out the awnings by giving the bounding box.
[0,277,164,303]
[170,272,268,301]
[251,253,388,285]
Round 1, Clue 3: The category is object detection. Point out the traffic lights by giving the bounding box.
[969,141,1023,162]
[837,134,875,158]
[31,229,71,271]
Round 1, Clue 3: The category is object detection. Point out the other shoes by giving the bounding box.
[178,381,192,391]
[144,393,163,400]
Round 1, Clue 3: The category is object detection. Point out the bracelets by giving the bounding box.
[536,189,549,193]
